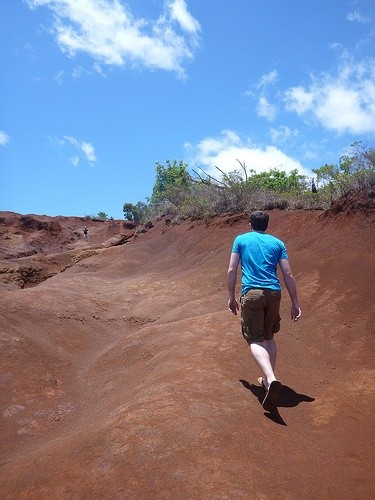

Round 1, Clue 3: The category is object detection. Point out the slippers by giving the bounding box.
[257,377,268,393]
[262,381,281,411]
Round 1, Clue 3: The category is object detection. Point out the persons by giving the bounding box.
[83,226,88,237]
[228,210,301,410]
